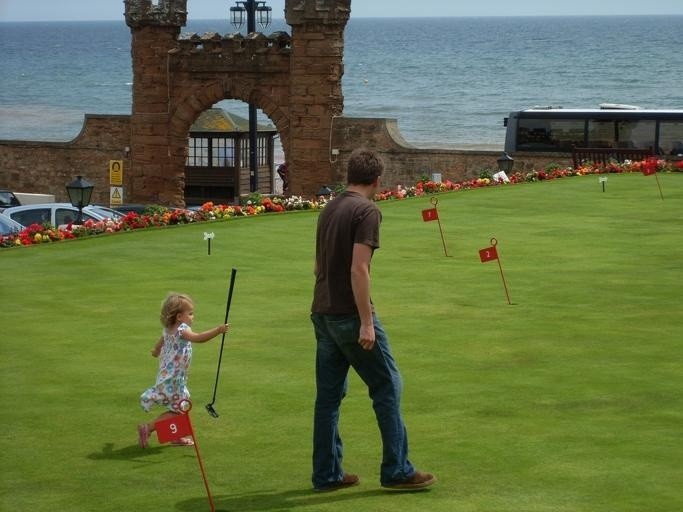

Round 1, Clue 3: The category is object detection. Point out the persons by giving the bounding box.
[311,148,437,490]
[277,163,288,195]
[138,293,232,449]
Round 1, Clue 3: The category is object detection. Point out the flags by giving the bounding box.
[422,208,438,222]
[643,166,655,176]
[479,247,498,263]
[154,414,193,444]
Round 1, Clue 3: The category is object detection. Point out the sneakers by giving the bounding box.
[313,474,359,490]
[171,436,193,444]
[136,424,148,449]
[380,470,436,488]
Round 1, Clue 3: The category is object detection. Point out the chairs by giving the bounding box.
[63,213,74,224]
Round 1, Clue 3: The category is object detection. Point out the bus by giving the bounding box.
[504,104,683,153]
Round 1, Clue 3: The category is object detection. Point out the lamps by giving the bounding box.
[330,149,340,164]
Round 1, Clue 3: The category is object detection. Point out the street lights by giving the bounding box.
[66,175,95,224]
[230,0,273,192]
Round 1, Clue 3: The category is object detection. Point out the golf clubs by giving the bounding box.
[205,268,236,419]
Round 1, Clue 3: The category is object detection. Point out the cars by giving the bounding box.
[0,191,202,236]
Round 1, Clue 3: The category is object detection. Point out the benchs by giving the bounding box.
[570,141,653,171]
